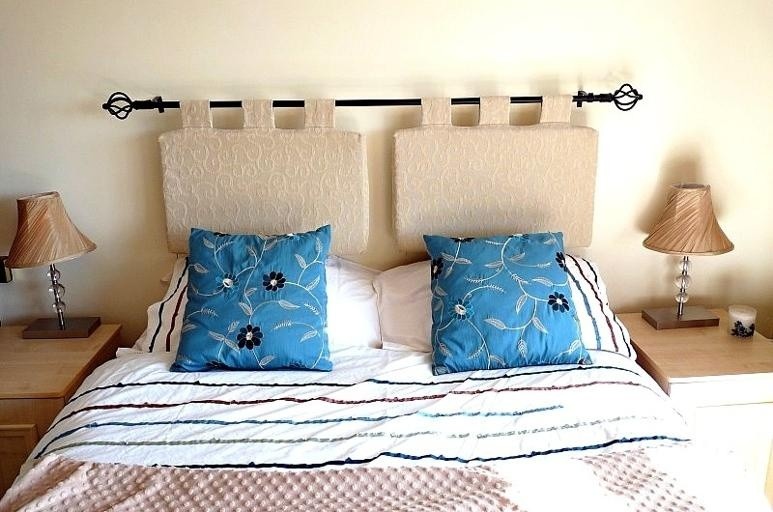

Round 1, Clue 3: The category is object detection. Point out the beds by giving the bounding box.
[0,84,707,512]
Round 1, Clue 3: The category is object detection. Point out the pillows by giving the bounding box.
[116,259,381,362]
[421,233,592,374]
[169,226,333,372]
[372,254,637,359]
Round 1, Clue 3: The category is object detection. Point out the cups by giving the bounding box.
[728,304,757,344]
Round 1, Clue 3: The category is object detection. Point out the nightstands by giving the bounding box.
[617,308,773,493]
[0,322,122,501]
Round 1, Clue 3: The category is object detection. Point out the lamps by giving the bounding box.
[642,183,735,330]
[4,191,101,338]
